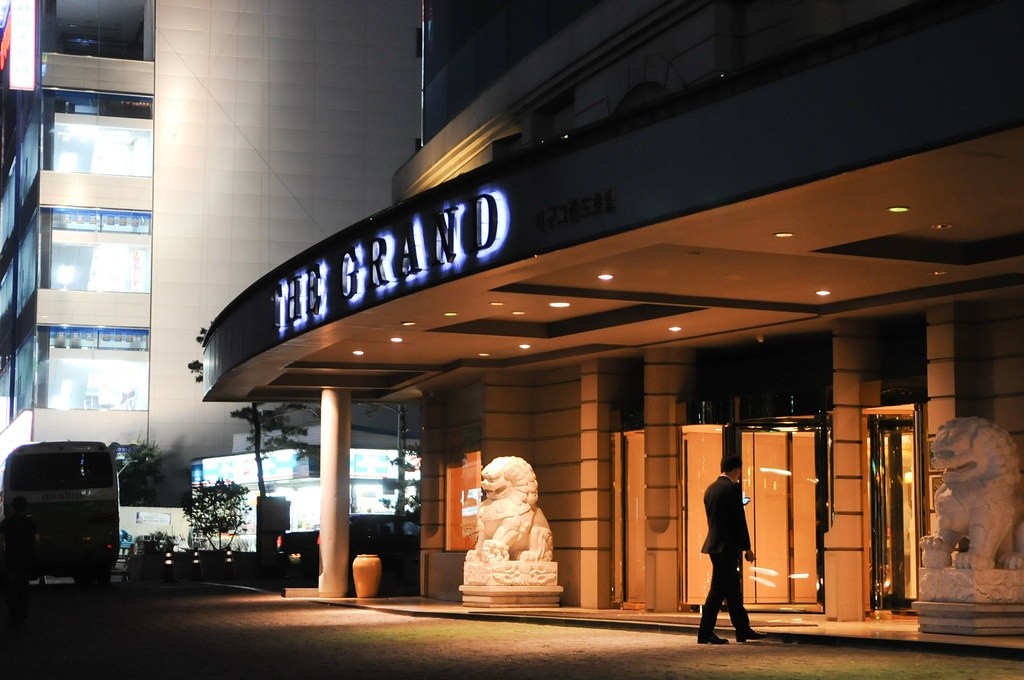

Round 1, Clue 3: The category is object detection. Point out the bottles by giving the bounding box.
[352,554,382,598]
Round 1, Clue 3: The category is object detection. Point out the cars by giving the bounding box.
[273,513,421,594]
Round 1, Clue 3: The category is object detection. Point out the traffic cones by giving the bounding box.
[189,547,208,583]
[161,545,181,583]
[221,546,241,581]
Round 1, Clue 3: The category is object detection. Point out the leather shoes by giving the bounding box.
[699,632,729,644]
[737,627,766,642]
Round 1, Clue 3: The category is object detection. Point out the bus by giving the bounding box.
[0,441,119,586]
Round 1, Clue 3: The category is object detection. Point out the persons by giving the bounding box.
[697,453,769,643]
[0,496,40,625]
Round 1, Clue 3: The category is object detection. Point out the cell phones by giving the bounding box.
[742,497,751,505]
